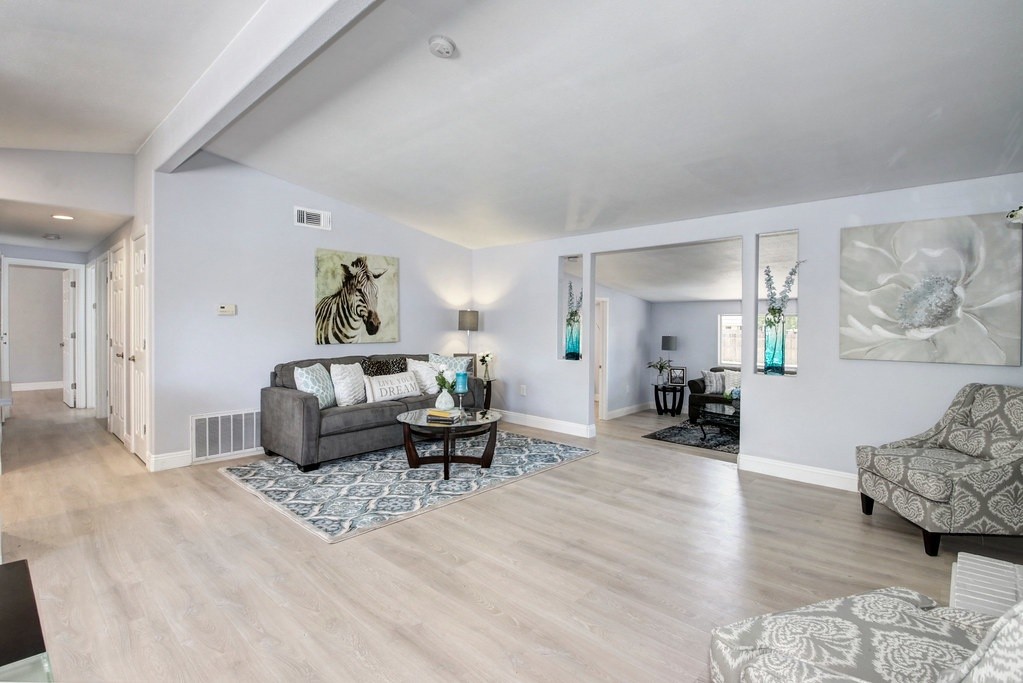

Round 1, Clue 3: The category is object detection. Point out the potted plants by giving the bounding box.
[480,354,490,380]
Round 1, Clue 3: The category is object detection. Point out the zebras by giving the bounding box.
[314,256,389,344]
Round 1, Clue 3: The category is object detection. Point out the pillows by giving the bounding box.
[361,357,407,376]
[294,363,336,410]
[363,371,424,403]
[701,370,725,394]
[330,362,367,406]
[428,354,474,377]
[406,358,447,395]
[723,369,741,396]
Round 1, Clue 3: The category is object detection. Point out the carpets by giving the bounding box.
[216,429,599,544]
[641,419,739,454]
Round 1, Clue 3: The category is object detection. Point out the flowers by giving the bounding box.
[764,259,806,308]
[567,281,583,314]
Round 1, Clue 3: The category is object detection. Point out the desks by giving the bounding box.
[655,384,686,417]
[396,408,502,480]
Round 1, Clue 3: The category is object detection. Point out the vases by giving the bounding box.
[435,370,456,411]
[764,308,785,376]
[565,312,580,361]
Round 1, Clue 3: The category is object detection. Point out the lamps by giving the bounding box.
[459,310,478,356]
[42,234,59,240]
[455,373,468,416]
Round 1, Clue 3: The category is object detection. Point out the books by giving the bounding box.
[427,413,460,424]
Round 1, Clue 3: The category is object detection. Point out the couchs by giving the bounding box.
[260,352,484,472]
[688,367,741,430]
[710,551,1022,683]
[856,383,1023,556]
